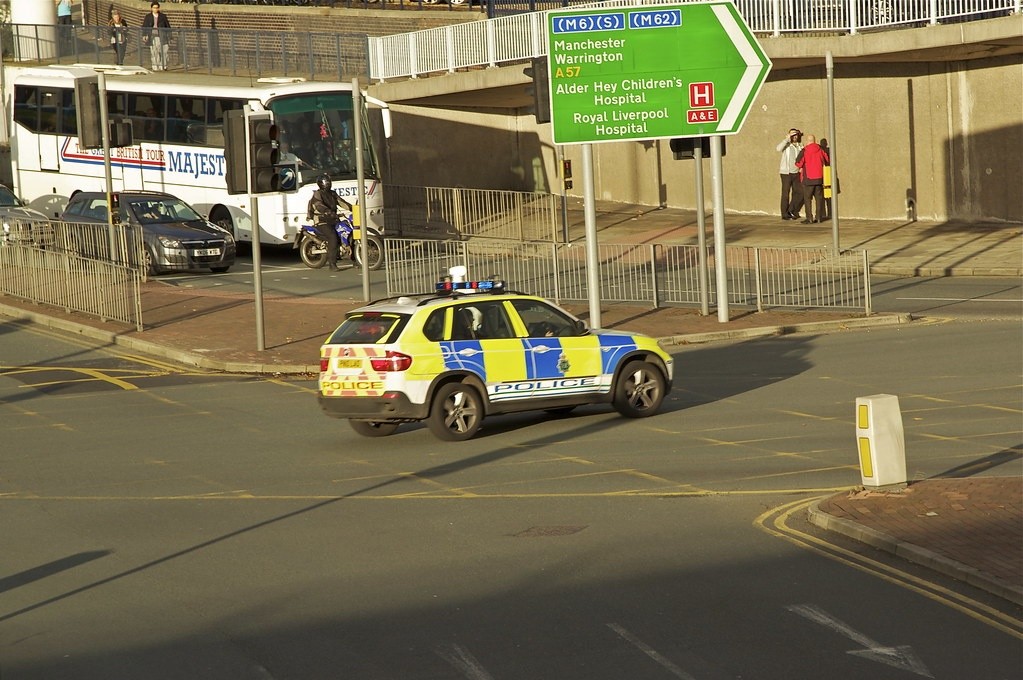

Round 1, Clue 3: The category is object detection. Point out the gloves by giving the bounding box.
[328,212,339,220]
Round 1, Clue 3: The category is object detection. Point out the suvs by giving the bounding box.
[57,190,237,277]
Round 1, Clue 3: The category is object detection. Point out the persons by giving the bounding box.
[311,173,352,272]
[151,203,162,219]
[460,308,475,328]
[142,108,158,137]
[795,135,830,224]
[141,1,172,72]
[55,0,73,44]
[776,128,803,220]
[181,110,193,122]
[280,141,302,166]
[107,10,128,65]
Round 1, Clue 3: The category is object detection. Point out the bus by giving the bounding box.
[0,60,394,258]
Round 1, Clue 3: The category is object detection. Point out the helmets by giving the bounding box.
[317,174,331,191]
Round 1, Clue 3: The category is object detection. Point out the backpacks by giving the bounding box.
[306,197,315,220]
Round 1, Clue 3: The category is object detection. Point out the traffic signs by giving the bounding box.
[545,0,775,146]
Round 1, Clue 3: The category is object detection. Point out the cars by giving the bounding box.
[316,266,672,444]
[1,183,57,253]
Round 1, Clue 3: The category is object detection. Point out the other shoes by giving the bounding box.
[814,219,821,223]
[783,210,800,220]
[801,220,812,224]
[329,263,341,272]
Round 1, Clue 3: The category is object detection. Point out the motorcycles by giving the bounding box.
[294,198,385,272]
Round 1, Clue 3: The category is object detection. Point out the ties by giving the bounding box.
[284,155,287,159]
